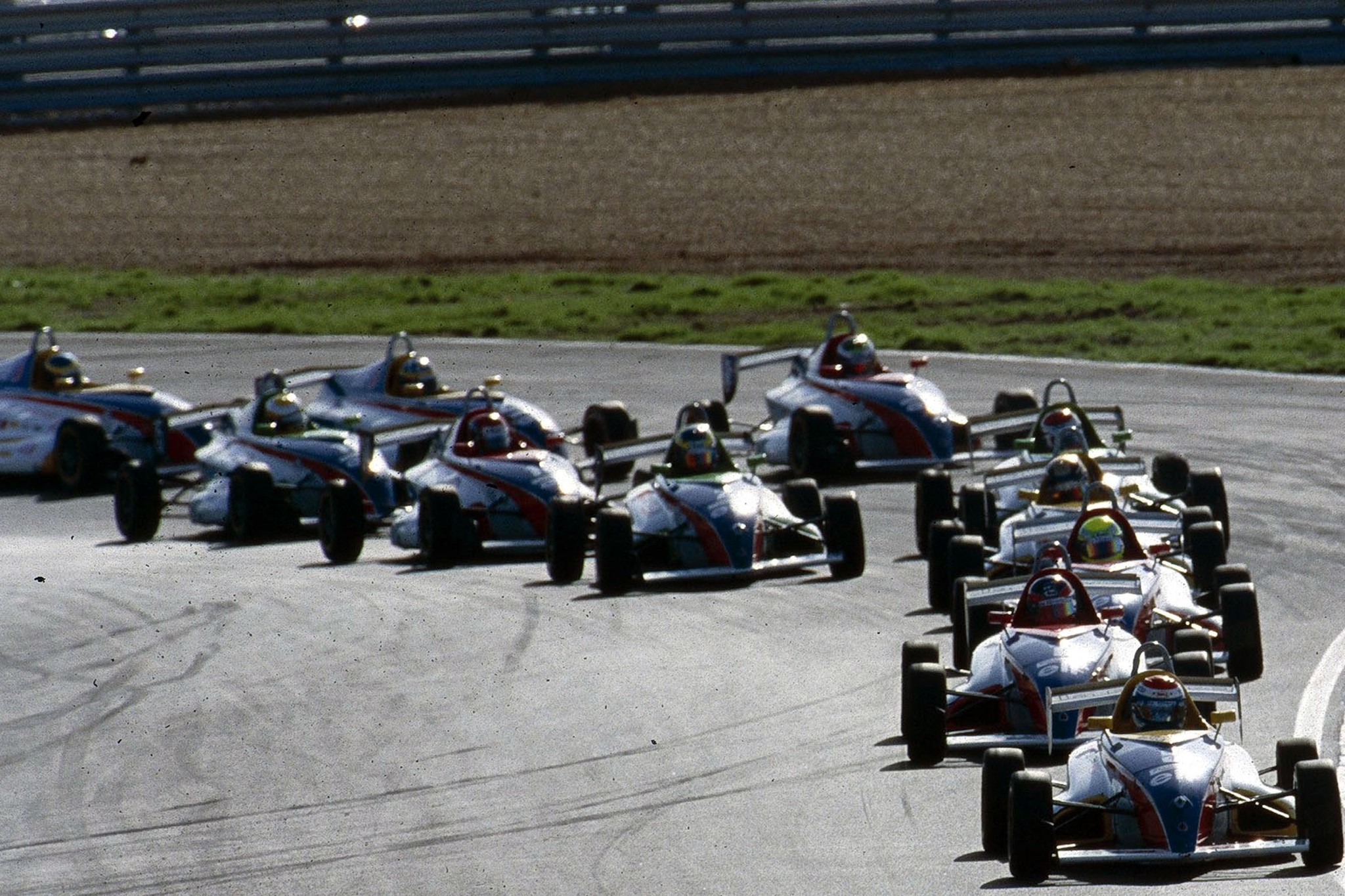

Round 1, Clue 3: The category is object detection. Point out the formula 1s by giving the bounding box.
[0,319,220,497]
[540,399,873,594]
[110,325,641,572]
[718,303,980,482]
[899,378,1345,888]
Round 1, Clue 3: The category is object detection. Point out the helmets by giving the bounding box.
[1078,515,1125,562]
[397,355,439,394]
[674,423,717,473]
[1047,454,1090,500]
[262,391,307,431]
[1022,576,1078,627]
[1131,673,1189,730]
[43,350,82,387]
[1042,410,1082,449]
[839,334,876,373]
[467,412,512,455]
